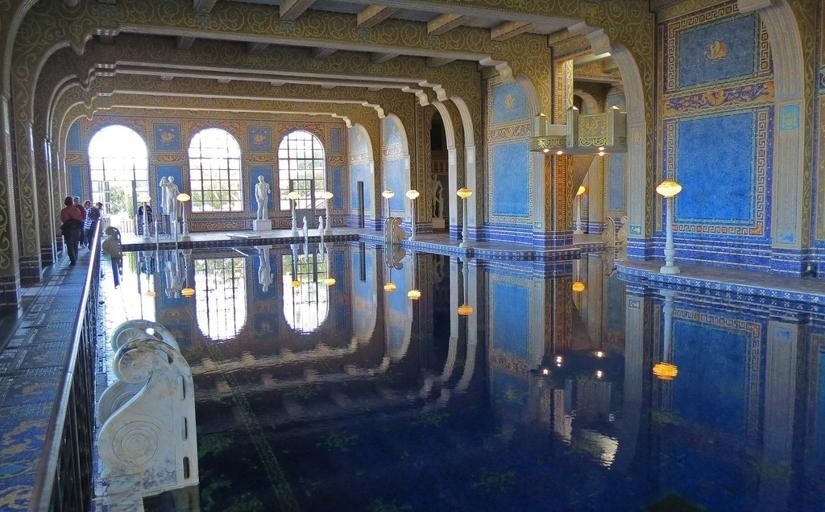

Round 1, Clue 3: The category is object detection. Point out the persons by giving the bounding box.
[165,254,187,299]
[159,176,184,224]
[73,196,87,248]
[83,201,103,251]
[82,199,91,211]
[257,248,275,293]
[139,252,156,279]
[137,202,153,236]
[59,196,83,266]
[253,175,272,220]
